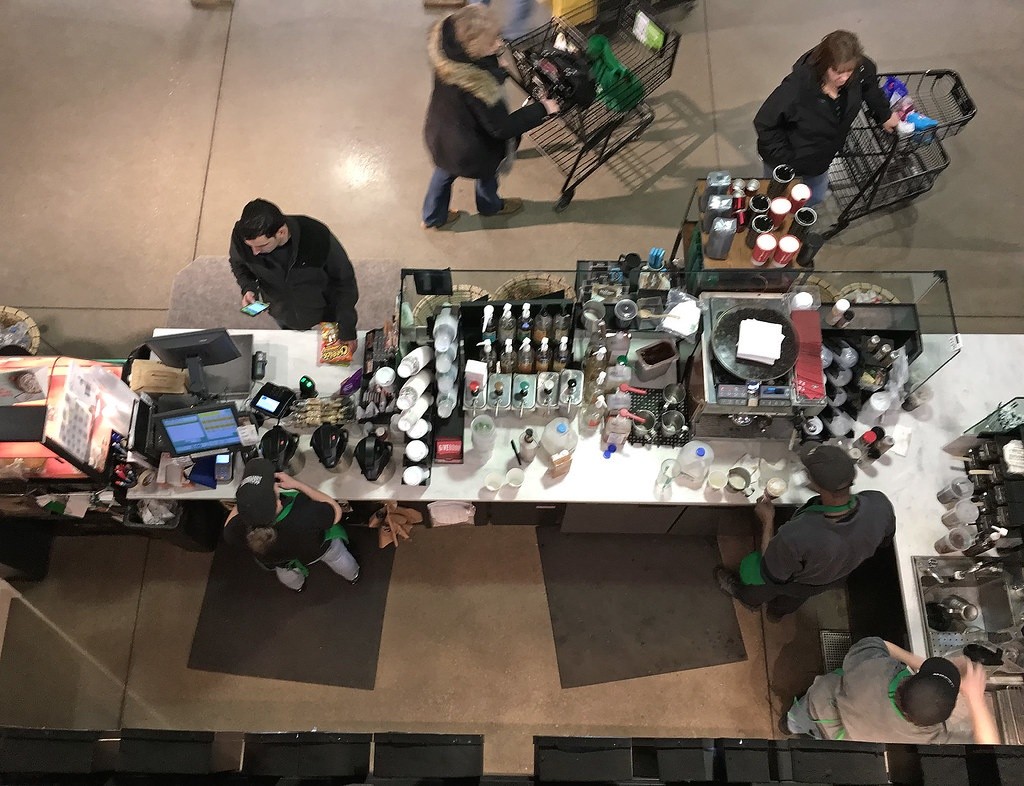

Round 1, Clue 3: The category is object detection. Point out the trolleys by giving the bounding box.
[494,0,683,212]
[819,67,979,240]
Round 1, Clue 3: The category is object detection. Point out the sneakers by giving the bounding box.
[421,210,463,230]
[482,197,523,218]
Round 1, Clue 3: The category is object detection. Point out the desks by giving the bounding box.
[125,326,1024,688]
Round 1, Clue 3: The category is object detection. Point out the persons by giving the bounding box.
[225,458,360,595]
[778,638,1001,744]
[755,29,900,207]
[228,198,359,355]
[418,4,559,228]
[715,442,896,625]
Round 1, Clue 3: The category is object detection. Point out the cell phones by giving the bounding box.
[240,300,270,318]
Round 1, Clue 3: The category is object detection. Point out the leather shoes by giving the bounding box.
[714,565,759,609]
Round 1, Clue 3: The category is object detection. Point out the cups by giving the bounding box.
[944,594,980,622]
[934,528,972,554]
[941,500,979,528]
[580,162,933,510]
[505,468,526,488]
[937,476,974,504]
[396,303,503,492]
[375,426,388,441]
[374,366,396,394]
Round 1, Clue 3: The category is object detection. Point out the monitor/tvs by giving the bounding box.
[151,401,252,458]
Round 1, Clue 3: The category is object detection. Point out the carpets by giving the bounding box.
[186,522,397,691]
[533,524,751,691]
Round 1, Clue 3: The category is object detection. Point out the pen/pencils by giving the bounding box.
[511,439,521,465]
[110,433,134,486]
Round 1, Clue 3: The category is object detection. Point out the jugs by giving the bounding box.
[309,421,352,473]
[352,431,395,485]
[259,424,306,476]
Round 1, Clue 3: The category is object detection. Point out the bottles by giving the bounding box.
[543,417,579,456]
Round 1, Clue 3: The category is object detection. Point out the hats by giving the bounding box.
[236,458,276,525]
[800,441,856,488]
